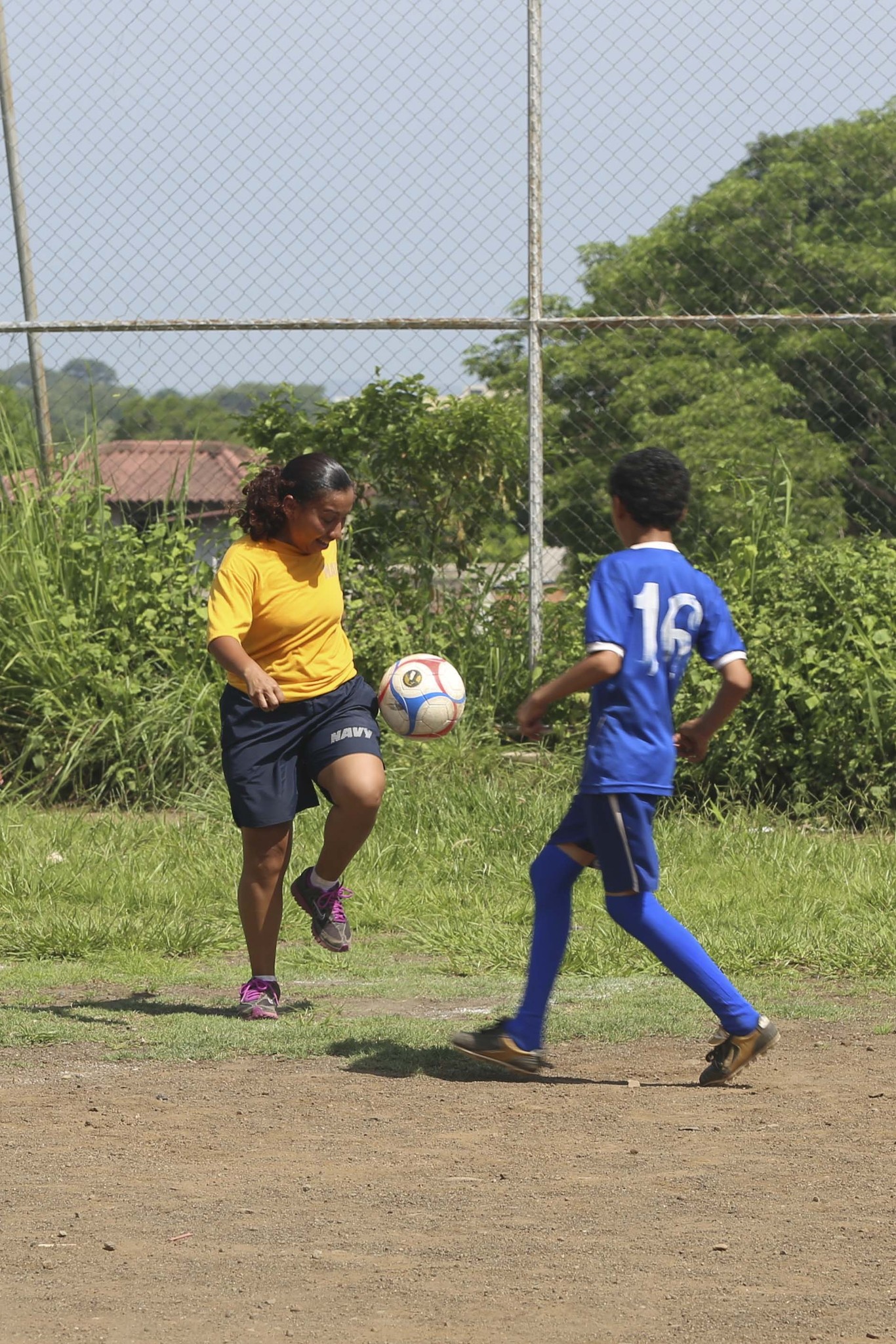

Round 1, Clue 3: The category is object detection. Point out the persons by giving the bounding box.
[204,451,385,1018]
[448,443,782,1084]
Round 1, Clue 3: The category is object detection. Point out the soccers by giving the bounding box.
[376,651,467,740]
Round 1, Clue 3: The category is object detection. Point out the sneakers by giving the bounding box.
[291,866,353,953]
[236,977,281,1021]
[452,1017,544,1075]
[699,1012,781,1087]
